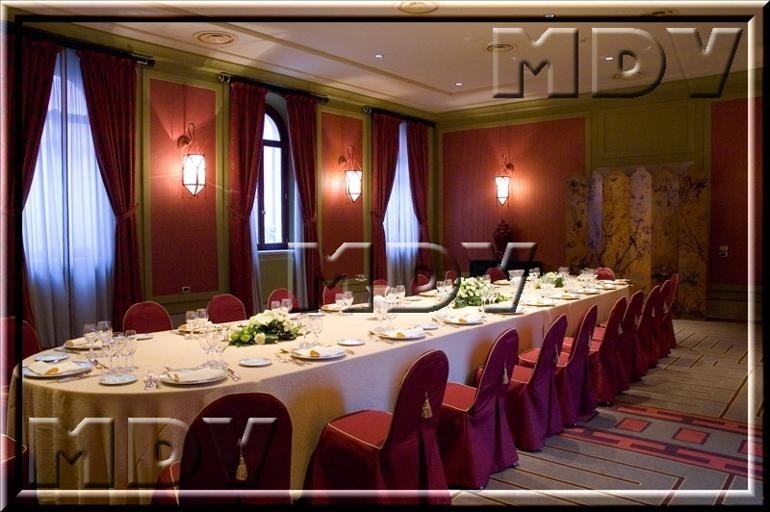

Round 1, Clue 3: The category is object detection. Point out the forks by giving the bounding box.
[143,367,161,390]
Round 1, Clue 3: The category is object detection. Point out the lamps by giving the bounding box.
[176,119,207,201]
[493,150,514,207]
[334,141,364,205]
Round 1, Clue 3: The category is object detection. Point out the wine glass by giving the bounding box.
[82,320,138,376]
[186,309,229,369]
[272,267,600,350]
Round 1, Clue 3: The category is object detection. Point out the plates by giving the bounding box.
[99,373,137,384]
[290,349,347,361]
[596,285,615,290]
[37,352,70,362]
[239,356,273,367]
[132,333,154,340]
[24,360,95,379]
[161,368,226,385]
[600,280,615,284]
[178,325,230,334]
[64,343,101,350]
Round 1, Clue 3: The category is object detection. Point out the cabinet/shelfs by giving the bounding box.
[469,259,537,280]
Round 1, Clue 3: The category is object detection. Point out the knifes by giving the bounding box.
[228,368,241,379]
